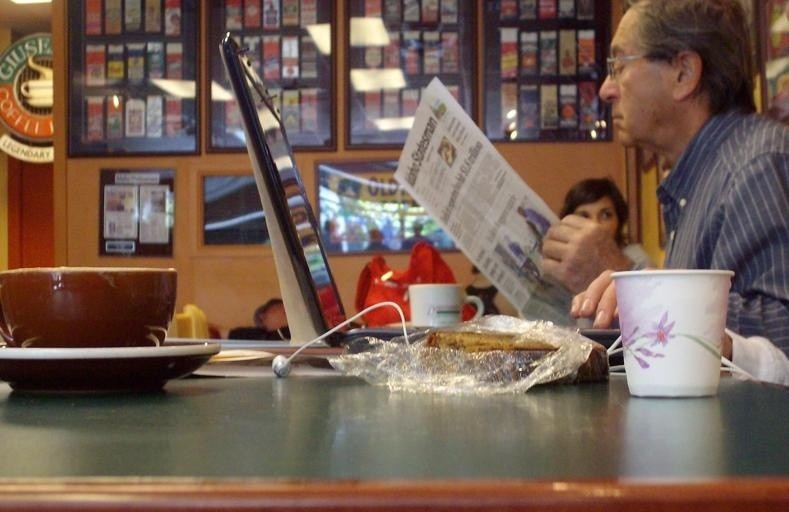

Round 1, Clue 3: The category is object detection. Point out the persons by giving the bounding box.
[364,229,391,252]
[252,293,292,342]
[568,266,788,390]
[563,50,575,70]
[504,236,540,278]
[537,2,789,359]
[401,223,435,251]
[320,220,344,253]
[557,175,651,269]
[327,178,378,252]
[517,206,550,240]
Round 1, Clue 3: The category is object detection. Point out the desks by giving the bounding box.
[0,361,789,511]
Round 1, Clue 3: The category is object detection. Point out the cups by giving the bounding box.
[20,80,53,100]
[610,268,737,397]
[0,268,177,346]
[407,283,485,327]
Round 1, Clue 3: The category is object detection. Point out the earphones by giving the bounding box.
[272,354,293,377]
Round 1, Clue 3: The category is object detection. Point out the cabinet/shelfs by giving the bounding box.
[51,0,788,338]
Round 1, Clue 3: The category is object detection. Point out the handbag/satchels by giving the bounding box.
[356,243,475,327]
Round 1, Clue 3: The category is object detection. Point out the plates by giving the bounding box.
[0,339,222,400]
[24,99,53,108]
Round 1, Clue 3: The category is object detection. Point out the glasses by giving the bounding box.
[607,54,646,81]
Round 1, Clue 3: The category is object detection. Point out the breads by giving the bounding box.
[429,331,610,384]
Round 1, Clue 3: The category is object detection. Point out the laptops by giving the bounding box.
[218,38,623,362]
